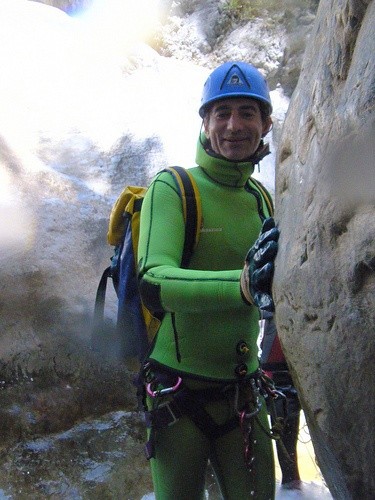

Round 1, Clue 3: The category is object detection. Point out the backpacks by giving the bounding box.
[91,163,275,372]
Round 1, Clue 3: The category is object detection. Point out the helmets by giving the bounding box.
[197,60,275,116]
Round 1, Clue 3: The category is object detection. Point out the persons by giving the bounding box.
[255,290,323,500]
[135,60,278,500]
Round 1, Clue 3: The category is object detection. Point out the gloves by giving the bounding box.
[240,218,279,313]
